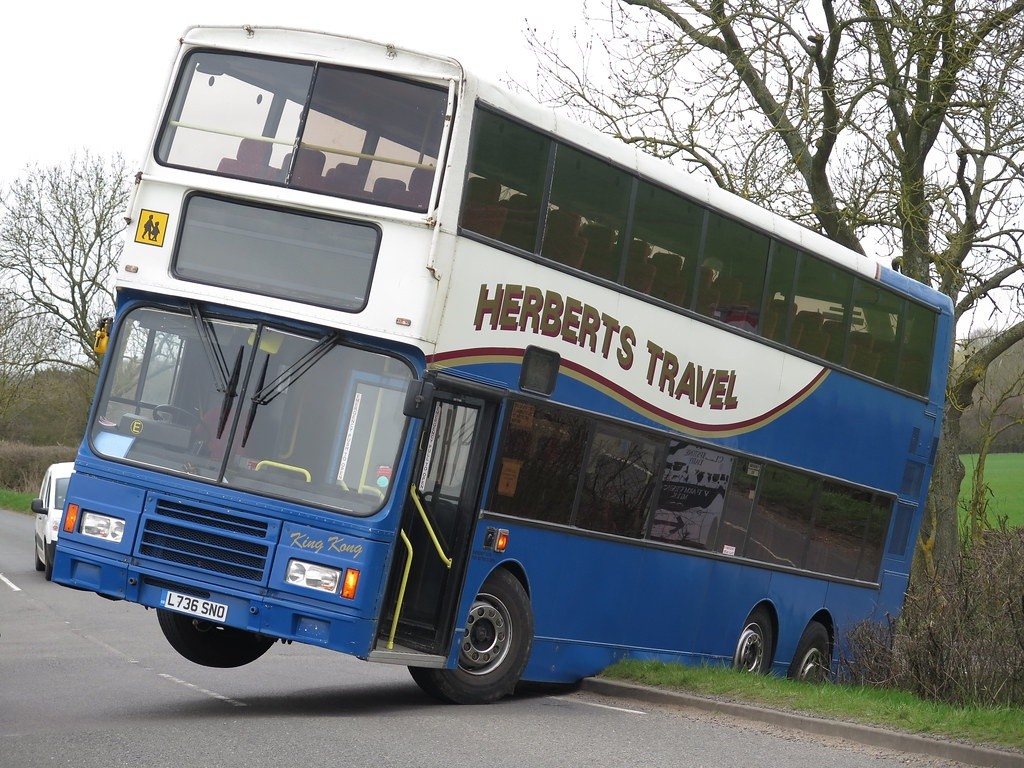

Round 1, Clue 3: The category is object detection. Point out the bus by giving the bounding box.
[52,26,956,704]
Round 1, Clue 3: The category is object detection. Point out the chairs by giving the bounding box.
[183,138,932,585]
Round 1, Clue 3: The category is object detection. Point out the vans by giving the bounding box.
[31,462,75,580]
